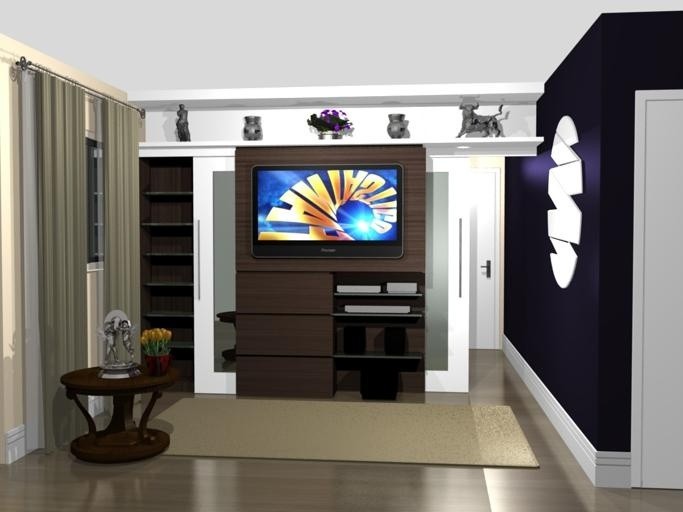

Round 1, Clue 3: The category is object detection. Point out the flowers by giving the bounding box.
[307,109,353,132]
[141,328,173,355]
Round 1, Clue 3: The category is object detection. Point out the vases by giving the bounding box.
[319,132,339,141]
[386,114,406,138]
[245,116,262,139]
[142,355,170,377]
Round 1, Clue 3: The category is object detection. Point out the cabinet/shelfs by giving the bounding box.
[138,157,194,392]
[235,271,425,402]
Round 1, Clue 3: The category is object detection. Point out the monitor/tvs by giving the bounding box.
[249,162,405,260]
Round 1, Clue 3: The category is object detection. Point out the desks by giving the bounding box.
[217,312,236,366]
[61,365,180,463]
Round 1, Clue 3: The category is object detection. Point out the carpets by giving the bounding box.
[148,397,540,469]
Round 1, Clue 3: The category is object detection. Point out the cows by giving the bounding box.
[456,100,503,137]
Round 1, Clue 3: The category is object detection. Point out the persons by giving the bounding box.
[104,322,120,364]
[119,320,136,362]
[176,104,190,141]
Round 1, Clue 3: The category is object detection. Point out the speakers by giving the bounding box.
[384,326,406,355]
[343,325,366,354]
[359,358,399,401]
